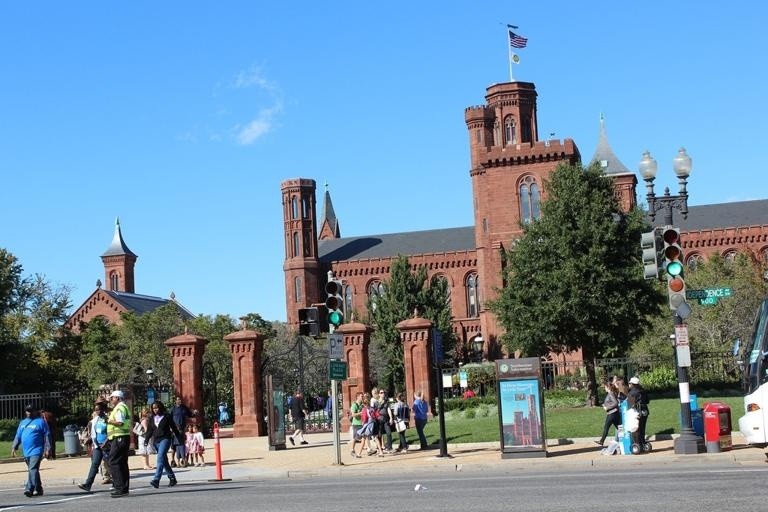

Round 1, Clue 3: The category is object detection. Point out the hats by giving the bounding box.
[24,404,35,411]
[628,377,640,385]
[93,396,110,405]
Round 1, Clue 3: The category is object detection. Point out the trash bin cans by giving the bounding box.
[704,401,732,453]
[679,394,704,437]
[64,432,80,455]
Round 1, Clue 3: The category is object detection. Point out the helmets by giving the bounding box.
[109,390,124,398]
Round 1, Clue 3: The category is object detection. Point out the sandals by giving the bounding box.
[145,466,155,470]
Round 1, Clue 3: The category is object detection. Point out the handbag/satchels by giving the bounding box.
[394,420,407,433]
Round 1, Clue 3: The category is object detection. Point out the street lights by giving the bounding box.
[145,366,155,413]
[669,330,678,379]
[473,331,487,398]
[635,144,708,457]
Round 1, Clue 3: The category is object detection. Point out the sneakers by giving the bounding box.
[290,436,295,446]
[592,440,604,447]
[168,477,178,488]
[110,490,131,498]
[23,490,33,499]
[301,440,309,445]
[77,483,92,492]
[350,447,409,459]
[100,478,111,485]
[32,489,45,496]
[170,461,209,469]
[148,480,161,488]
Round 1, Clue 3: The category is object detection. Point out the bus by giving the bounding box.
[737,295,768,461]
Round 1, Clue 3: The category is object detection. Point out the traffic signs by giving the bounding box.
[685,288,733,299]
[325,333,345,360]
[329,360,348,381]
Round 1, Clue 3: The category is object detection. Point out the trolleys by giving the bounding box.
[628,399,655,455]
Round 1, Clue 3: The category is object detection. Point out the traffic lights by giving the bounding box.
[324,278,344,327]
[638,225,662,283]
[295,304,323,339]
[660,224,687,313]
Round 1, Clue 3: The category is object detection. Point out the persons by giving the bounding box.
[11,403,52,496]
[219,401,230,429]
[39,406,58,461]
[76,390,206,496]
[289,387,429,457]
[592,374,649,450]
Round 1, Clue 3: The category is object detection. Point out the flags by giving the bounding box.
[508,30,526,48]
